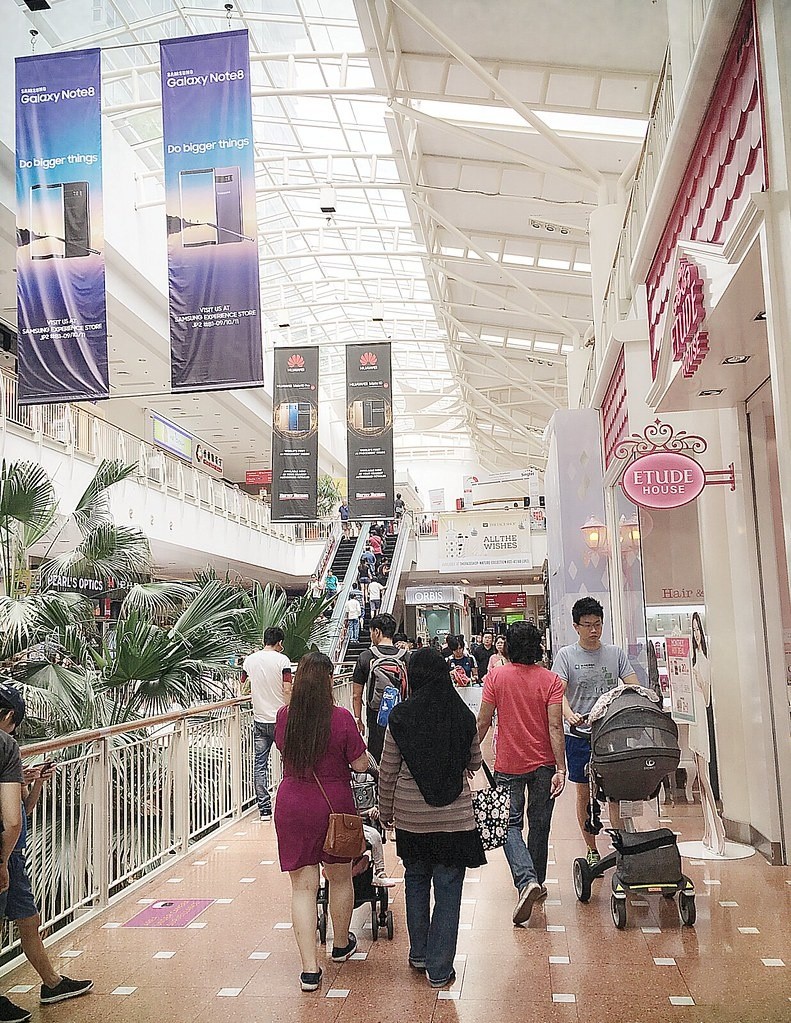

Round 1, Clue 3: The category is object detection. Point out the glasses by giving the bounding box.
[576,623,601,630]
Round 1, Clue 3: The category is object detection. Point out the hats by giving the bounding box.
[0,684,25,735]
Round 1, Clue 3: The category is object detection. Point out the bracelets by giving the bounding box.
[556,770,566,775]
[355,716,361,720]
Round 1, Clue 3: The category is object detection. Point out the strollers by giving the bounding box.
[314,768,394,944]
[568,689,697,929]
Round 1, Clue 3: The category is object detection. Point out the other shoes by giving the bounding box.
[408,958,426,967]
[370,871,395,887]
[430,968,455,988]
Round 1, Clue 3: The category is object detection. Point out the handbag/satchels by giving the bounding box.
[469,759,511,849]
[323,813,363,858]
[605,827,683,884]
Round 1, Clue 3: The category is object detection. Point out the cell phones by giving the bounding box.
[31,761,54,778]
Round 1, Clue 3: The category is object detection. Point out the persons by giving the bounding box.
[391,632,509,687]
[394,493,431,536]
[0,681,94,1023]
[51,406,74,444]
[468,621,567,923]
[335,500,352,540]
[352,612,413,766]
[307,569,338,625]
[378,646,487,987]
[147,447,165,481]
[344,524,392,644]
[241,626,292,821]
[550,597,640,879]
[505,502,518,511]
[688,611,726,856]
[274,652,369,992]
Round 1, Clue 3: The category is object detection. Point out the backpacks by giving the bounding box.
[366,646,409,712]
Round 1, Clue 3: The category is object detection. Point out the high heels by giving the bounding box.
[702,830,713,848]
[716,839,725,856]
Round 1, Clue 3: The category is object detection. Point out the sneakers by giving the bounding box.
[332,932,357,961]
[586,845,604,878]
[0,996,32,1023]
[537,884,547,899]
[513,882,542,925]
[41,975,94,1005]
[299,966,323,991]
[260,808,272,820]
